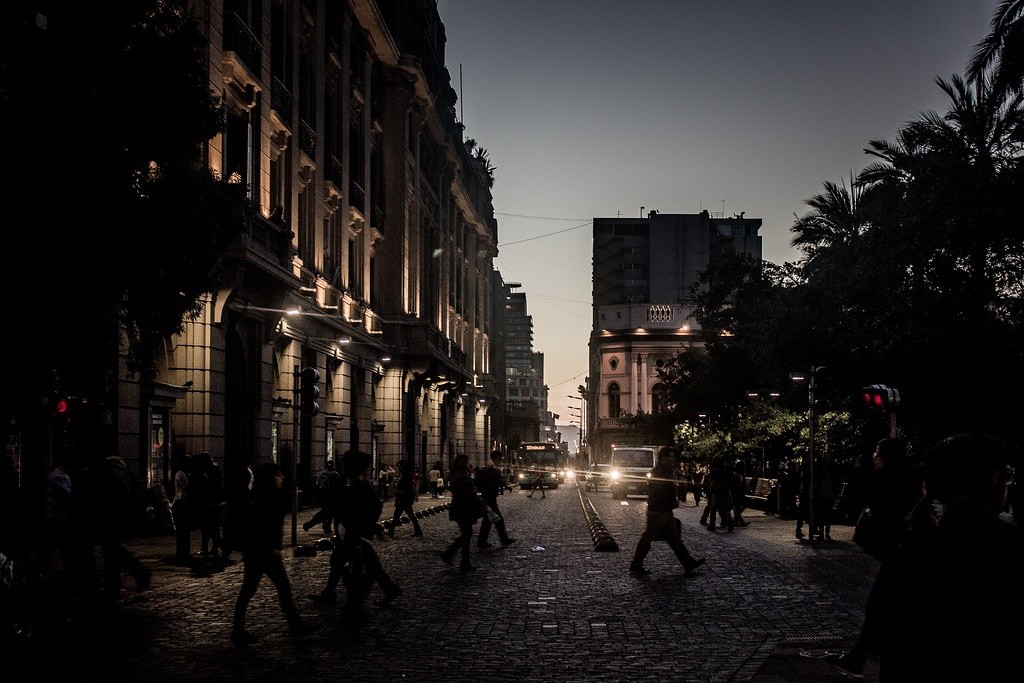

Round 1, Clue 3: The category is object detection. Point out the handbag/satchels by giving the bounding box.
[436,477,444,488]
[483,505,500,523]
[479,499,489,517]
[652,516,681,541]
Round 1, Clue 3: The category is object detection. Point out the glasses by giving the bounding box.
[872,452,883,459]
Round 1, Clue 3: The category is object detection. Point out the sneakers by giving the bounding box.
[825,655,864,678]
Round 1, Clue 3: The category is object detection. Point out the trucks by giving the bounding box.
[610,448,655,500]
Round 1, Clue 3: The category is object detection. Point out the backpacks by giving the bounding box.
[475,466,491,495]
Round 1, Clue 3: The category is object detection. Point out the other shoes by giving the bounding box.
[440,551,451,564]
[409,532,421,537]
[631,567,650,575]
[540,496,546,499]
[510,488,512,493]
[501,539,515,546]
[382,586,401,604]
[383,531,394,537]
[499,493,504,495]
[685,557,705,572]
[477,543,492,548]
[308,591,337,607]
[229,631,256,642]
[700,515,751,532]
[459,563,477,572]
[289,619,319,640]
[527,495,532,497]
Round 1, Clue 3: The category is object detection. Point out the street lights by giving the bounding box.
[568,396,585,446]
[789,366,826,541]
[698,412,720,424]
[747,388,782,479]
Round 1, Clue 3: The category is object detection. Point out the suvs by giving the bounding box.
[586,464,608,482]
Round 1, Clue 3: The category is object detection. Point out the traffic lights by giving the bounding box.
[47,390,107,419]
[861,385,904,409]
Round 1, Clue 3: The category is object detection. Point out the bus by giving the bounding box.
[517,440,558,490]
[558,450,565,484]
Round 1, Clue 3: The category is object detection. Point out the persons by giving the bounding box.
[860,434,1024,683]
[826,436,927,677]
[88,441,546,644]
[678,438,831,540]
[631,446,707,578]
